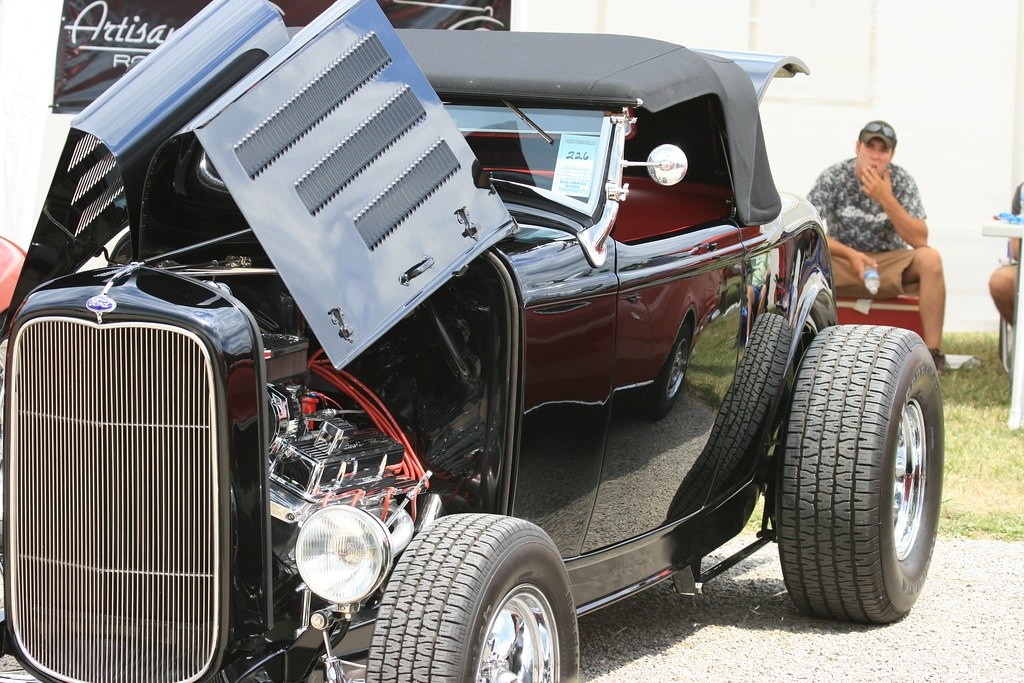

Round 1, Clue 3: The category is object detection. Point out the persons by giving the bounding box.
[744,252,773,347]
[806,121,949,373]
[988,181,1024,328]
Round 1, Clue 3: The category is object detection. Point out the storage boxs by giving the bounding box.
[834,292,925,342]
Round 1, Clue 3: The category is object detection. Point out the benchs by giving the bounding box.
[610,175,733,242]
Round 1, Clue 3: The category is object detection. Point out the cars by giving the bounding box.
[0,0,946,682]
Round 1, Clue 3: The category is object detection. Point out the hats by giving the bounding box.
[859,120,898,151]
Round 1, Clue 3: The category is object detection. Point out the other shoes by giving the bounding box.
[929,349,941,371]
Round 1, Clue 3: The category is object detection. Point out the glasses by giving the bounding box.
[864,123,894,139]
